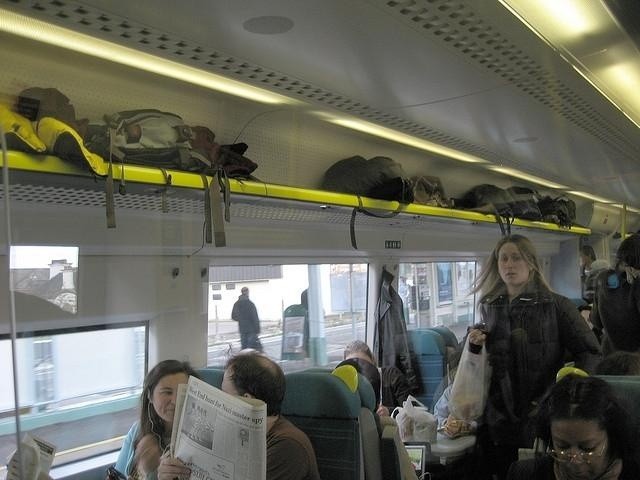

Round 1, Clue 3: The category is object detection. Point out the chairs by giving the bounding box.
[189,298,640,480]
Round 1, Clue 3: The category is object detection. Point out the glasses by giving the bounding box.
[545,435,607,464]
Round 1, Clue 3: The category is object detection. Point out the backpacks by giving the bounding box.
[13,86,259,186]
[320,155,576,251]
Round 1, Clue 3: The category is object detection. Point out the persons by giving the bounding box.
[231,286,261,348]
[104,359,201,480]
[356,358,417,479]
[431,224,639,480]
[344,338,413,419]
[398,276,411,324]
[156,353,322,479]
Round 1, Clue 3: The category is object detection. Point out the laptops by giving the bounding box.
[403,442,432,480]
